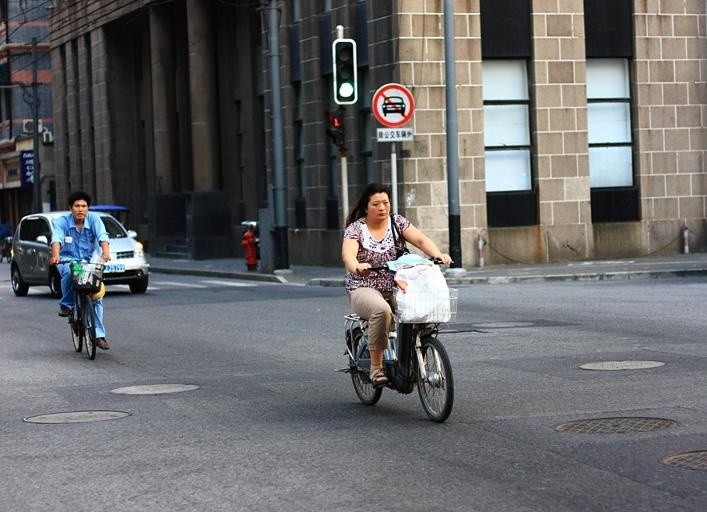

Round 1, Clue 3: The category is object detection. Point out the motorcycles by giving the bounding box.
[333,256,454,422]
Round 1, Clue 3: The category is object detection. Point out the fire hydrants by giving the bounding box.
[241,226,259,271]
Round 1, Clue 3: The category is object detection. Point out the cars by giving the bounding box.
[9,212,150,295]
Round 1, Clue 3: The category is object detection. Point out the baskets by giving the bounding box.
[391,285,459,324]
[70,262,106,294]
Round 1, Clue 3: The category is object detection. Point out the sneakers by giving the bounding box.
[369,368,388,382]
[59,305,69,317]
[96,338,110,350]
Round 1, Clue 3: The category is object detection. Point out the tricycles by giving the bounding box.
[1,223,12,263]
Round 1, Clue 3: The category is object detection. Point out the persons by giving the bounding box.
[0,217,13,257]
[341,184,454,384]
[48,191,111,350]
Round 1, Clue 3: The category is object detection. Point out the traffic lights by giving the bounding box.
[331,38,359,106]
[325,110,346,153]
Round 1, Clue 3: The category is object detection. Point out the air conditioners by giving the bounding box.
[22,118,43,134]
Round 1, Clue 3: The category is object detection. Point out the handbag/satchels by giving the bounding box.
[396,246,410,260]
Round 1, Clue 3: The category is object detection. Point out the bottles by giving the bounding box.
[72,259,88,278]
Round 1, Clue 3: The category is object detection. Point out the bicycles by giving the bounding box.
[50,256,111,360]
